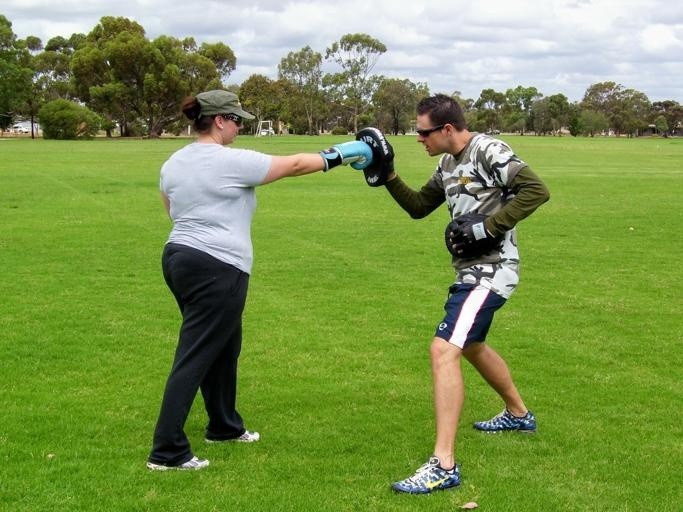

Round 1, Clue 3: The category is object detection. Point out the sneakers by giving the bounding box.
[203,430,262,443]
[391,455,463,493]
[145,455,210,471]
[473,406,537,431]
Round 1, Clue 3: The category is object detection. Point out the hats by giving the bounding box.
[193,90,256,121]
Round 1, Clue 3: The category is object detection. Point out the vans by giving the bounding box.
[7,125,28,133]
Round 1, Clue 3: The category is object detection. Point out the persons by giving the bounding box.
[354,93,550,494]
[144,89,374,474]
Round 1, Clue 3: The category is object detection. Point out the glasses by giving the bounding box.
[220,115,243,127]
[416,124,447,140]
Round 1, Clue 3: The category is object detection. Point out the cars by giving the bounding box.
[486,127,500,135]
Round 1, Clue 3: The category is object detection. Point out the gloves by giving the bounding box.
[320,138,374,175]
